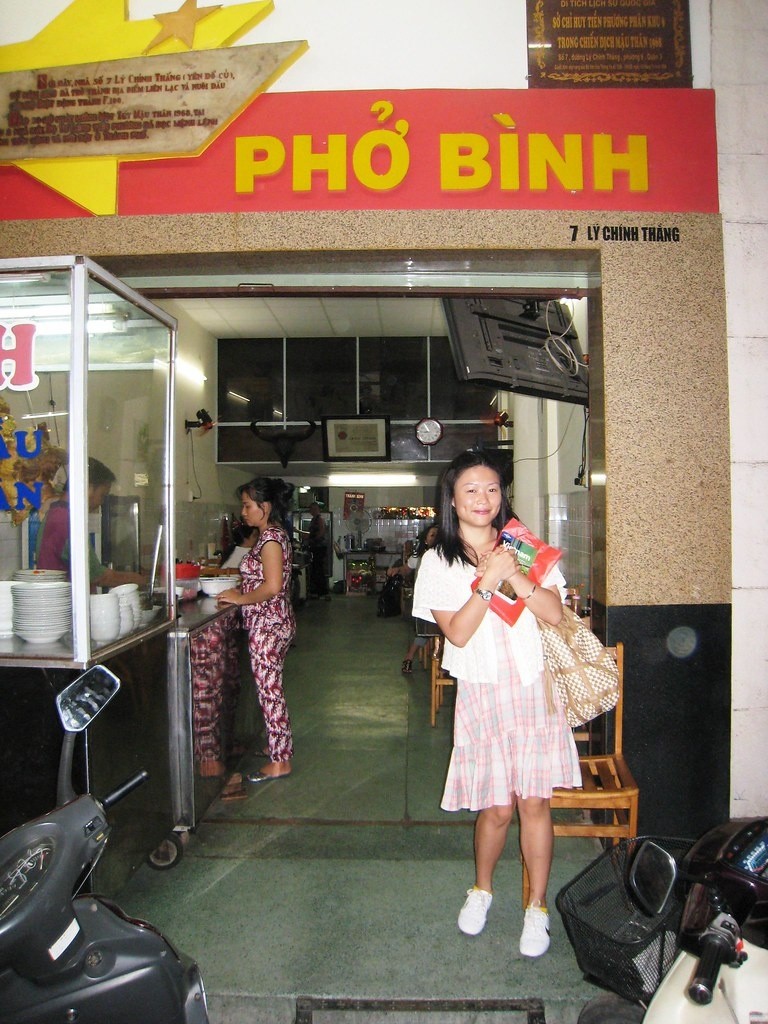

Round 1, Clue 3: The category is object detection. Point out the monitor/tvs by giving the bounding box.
[441,291,590,405]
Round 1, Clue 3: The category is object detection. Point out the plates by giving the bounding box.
[11,568,72,644]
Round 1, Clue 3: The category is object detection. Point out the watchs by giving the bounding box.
[475,588,494,600]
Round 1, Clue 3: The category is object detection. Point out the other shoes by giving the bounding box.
[401,659,412,673]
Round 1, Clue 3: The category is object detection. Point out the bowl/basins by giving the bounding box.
[199,578,239,597]
[90,582,162,646]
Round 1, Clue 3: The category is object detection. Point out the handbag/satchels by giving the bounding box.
[538,605,619,728]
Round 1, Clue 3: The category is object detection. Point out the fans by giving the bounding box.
[346,508,372,534]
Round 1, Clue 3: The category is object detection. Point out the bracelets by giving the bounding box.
[520,585,536,600]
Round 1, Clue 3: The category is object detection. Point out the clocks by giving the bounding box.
[415,417,443,445]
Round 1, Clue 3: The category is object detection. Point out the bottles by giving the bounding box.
[687,907,743,1005]
[570,584,591,618]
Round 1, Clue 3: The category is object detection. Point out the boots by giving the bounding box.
[253,749,271,758]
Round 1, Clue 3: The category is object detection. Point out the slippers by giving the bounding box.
[246,769,291,783]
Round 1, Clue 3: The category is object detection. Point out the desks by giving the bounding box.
[416,618,457,727]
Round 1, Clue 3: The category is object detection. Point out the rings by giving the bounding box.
[222,595,224,597]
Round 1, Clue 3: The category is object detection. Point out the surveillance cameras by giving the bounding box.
[493,410,508,426]
[197,408,213,431]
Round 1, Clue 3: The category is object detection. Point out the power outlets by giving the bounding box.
[189,491,193,502]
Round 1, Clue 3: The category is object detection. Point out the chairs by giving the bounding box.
[522,643,639,909]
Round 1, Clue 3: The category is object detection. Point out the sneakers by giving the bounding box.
[519,900,550,956]
[458,887,492,935]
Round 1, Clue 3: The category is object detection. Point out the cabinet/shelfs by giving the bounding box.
[343,551,403,596]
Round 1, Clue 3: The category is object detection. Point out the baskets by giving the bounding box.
[553,837,697,1001]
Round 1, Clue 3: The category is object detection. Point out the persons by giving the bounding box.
[35,456,147,593]
[190,611,246,784]
[305,501,327,595]
[386,525,439,673]
[411,451,583,957]
[214,477,296,783]
[220,525,258,570]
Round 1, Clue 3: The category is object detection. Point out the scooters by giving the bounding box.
[556,813,768,1024]
[1,661,216,1024]
